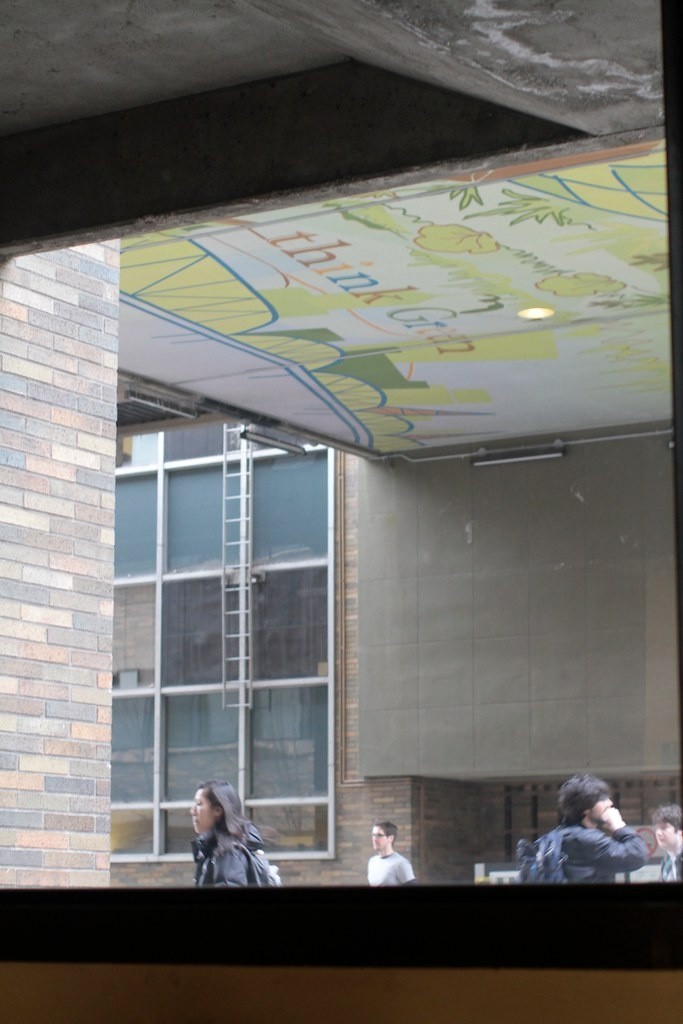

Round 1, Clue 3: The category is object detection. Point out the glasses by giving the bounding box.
[369,834,387,839]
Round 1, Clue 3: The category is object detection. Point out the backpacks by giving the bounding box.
[198,842,282,886]
[515,818,582,884]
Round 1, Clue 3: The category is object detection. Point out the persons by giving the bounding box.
[368,821,415,887]
[190,780,281,886]
[654,805,683,882]
[519,772,648,884]
